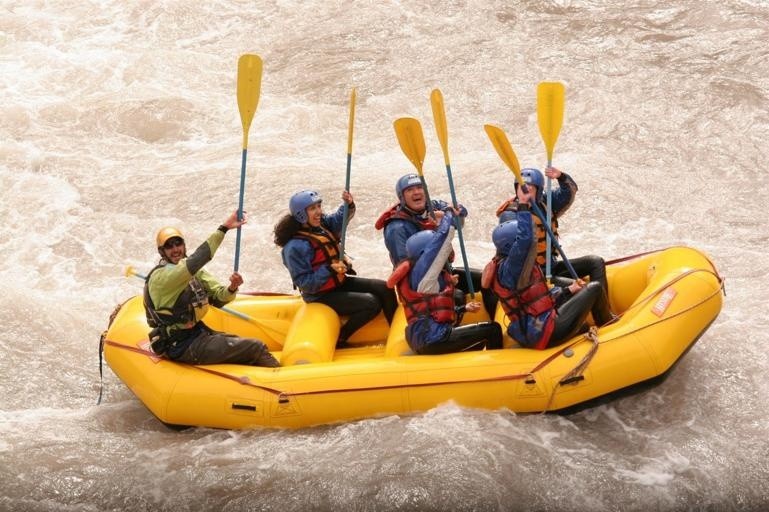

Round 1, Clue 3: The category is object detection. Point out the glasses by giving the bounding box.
[160,238,184,250]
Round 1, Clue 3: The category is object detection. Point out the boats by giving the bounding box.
[104,247,723,430]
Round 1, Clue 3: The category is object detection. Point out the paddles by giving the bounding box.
[536,80,566,285]
[338,88,357,273]
[121,264,291,345]
[392,116,454,275]
[428,88,477,305]
[483,123,581,284]
[233,53,263,273]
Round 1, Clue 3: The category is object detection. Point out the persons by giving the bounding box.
[387,200,504,356]
[142,208,281,369]
[273,189,398,348]
[375,174,468,269]
[497,165,609,294]
[482,184,619,351]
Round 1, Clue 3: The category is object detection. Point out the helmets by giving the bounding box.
[289,189,324,225]
[514,167,546,202]
[405,229,437,259]
[491,218,519,256]
[157,226,184,252]
[395,173,423,207]
